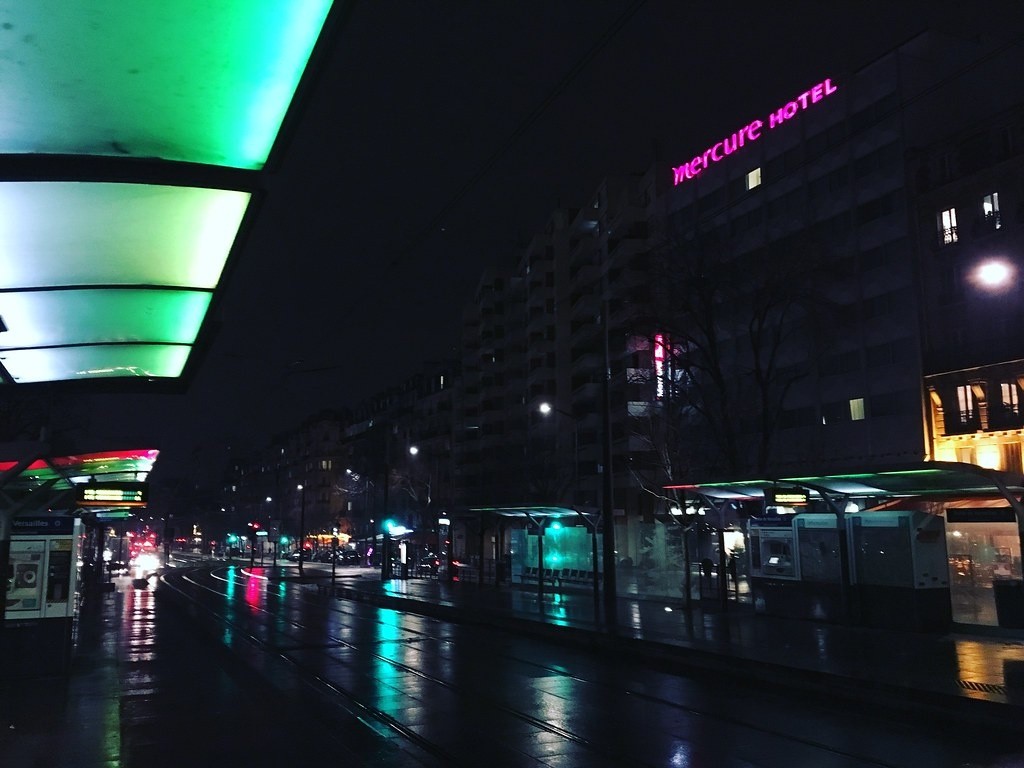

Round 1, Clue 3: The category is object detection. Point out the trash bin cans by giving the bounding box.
[993,576,1024,626]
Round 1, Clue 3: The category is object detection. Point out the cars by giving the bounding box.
[414,557,460,578]
[126,530,361,568]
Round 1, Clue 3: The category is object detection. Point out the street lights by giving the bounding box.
[380,410,418,583]
[538,400,580,512]
[296,457,305,579]
